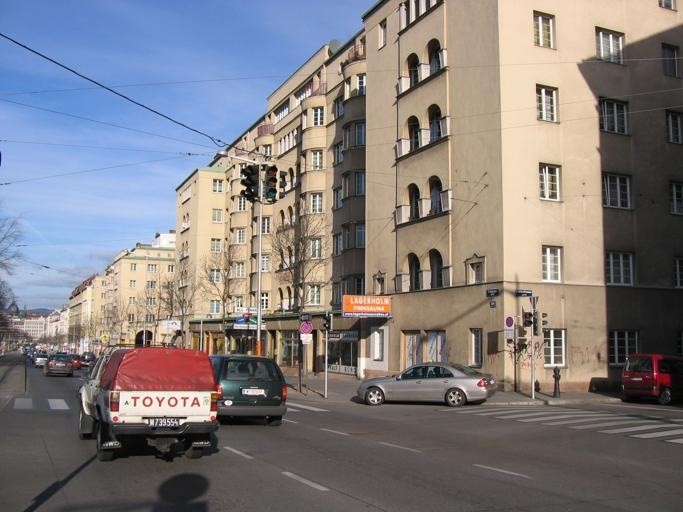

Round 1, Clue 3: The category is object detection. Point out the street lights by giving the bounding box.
[216,149,266,356]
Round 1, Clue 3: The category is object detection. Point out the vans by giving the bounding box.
[205,353,288,426]
[621,352,683,406]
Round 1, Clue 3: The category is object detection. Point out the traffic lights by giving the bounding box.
[300,312,312,321]
[279,170,287,200]
[264,165,277,203]
[540,312,549,333]
[521,311,533,328]
[322,314,333,331]
[246,164,258,198]
[533,310,540,335]
[240,168,250,201]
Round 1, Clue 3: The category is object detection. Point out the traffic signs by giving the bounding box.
[232,307,267,332]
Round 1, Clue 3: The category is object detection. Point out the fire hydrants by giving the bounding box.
[550,365,561,398]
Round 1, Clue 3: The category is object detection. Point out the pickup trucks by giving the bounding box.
[75,342,224,462]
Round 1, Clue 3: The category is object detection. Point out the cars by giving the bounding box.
[356,360,498,409]
[22,345,97,377]
